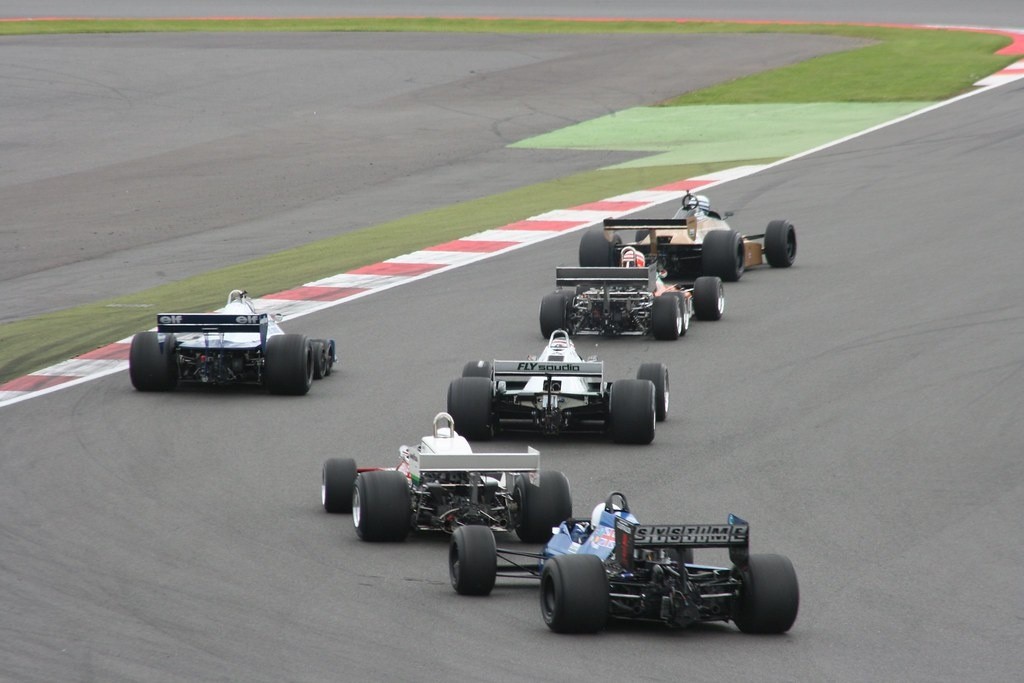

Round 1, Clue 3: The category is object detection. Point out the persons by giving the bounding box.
[587,503,623,535]
[624,250,647,272]
[549,336,576,354]
[689,195,710,220]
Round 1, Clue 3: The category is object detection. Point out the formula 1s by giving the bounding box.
[320,412,573,542]
[448,328,670,446]
[446,490,801,636]
[130,290,337,393]
[541,246,726,344]
[579,189,797,286]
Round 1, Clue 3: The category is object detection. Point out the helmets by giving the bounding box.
[689,195,710,211]
[624,250,645,267]
[591,502,621,530]
